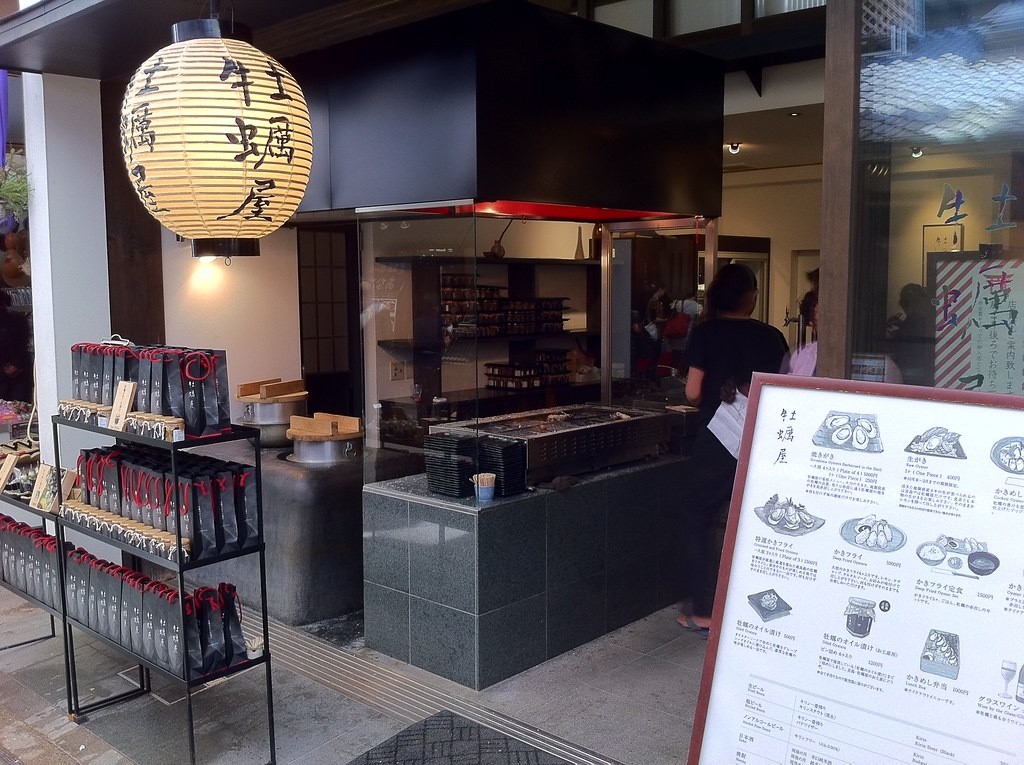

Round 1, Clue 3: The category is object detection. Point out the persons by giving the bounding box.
[0,221,33,405]
[675,265,794,637]
[802,267,819,337]
[630,280,665,380]
[670,289,704,376]
[888,283,925,368]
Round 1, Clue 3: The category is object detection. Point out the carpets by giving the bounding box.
[347,710,576,765]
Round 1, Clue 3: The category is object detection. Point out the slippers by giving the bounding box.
[675,616,709,637]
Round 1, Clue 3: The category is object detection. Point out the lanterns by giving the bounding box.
[119,18,314,258]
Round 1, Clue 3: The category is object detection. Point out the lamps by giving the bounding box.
[912,147,923,158]
[728,142,740,154]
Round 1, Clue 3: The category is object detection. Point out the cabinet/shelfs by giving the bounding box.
[0,494,144,720]
[375,255,601,412]
[51,414,277,765]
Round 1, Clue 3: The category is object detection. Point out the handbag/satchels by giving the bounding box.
[0,342,258,679]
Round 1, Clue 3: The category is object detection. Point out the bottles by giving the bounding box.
[491,240,505,257]
[575,226,584,259]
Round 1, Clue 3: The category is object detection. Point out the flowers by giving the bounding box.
[0,161,36,223]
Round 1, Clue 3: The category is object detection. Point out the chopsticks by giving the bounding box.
[470,472,496,485]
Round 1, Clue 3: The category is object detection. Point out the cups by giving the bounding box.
[474,485,495,502]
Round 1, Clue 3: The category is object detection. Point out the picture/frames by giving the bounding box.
[922,224,965,287]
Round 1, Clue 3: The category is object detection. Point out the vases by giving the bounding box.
[0,200,7,220]
